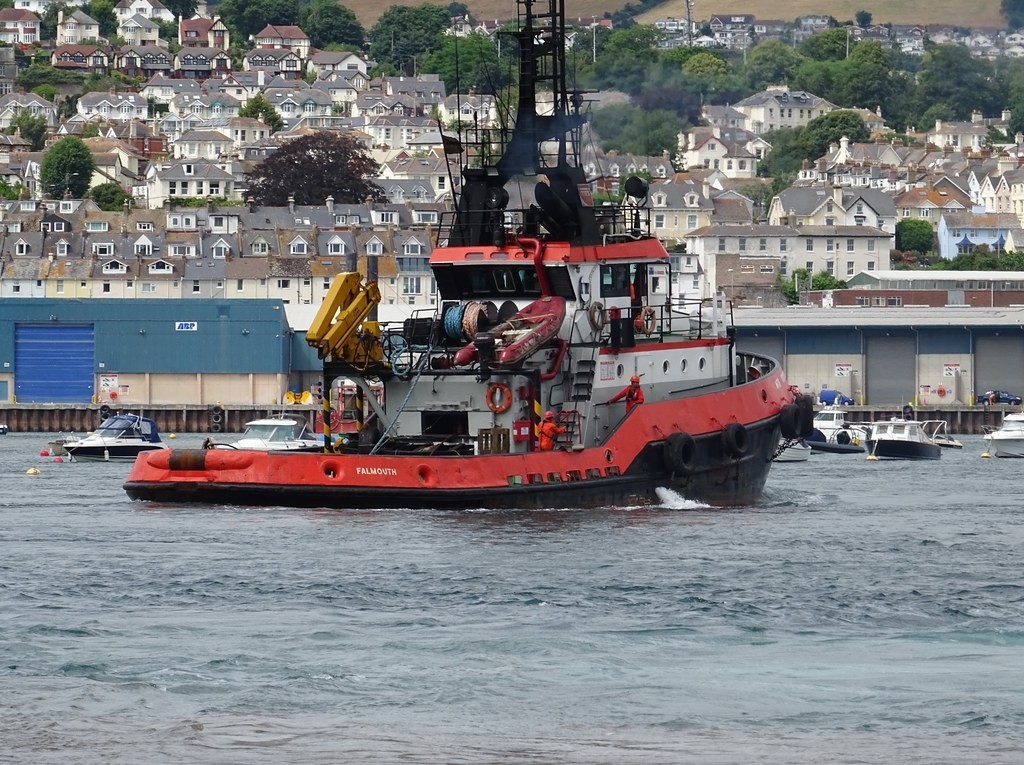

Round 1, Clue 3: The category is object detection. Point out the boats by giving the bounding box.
[805,424,866,454]
[920,420,963,449]
[48,434,80,456]
[0,425,8,435]
[63,413,169,464]
[863,417,942,460]
[123,0,814,512]
[981,413,1024,459]
[773,436,812,461]
[217,417,336,453]
[813,405,867,441]
[455,294,566,369]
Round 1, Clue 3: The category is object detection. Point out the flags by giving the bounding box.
[443,135,465,155]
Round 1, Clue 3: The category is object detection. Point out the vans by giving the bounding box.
[819,390,854,406]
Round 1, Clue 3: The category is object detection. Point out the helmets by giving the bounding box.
[631,376,640,382]
[545,411,554,418]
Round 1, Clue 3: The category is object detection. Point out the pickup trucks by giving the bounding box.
[976,390,1022,406]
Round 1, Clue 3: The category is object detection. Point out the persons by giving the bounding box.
[989,394,995,404]
[536,411,567,453]
[604,376,645,414]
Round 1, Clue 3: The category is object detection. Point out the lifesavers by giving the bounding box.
[589,302,608,331]
[663,432,699,476]
[780,402,804,440]
[639,306,656,335]
[100,405,111,422]
[486,382,514,414]
[719,421,750,461]
[210,405,223,432]
[794,394,815,438]
